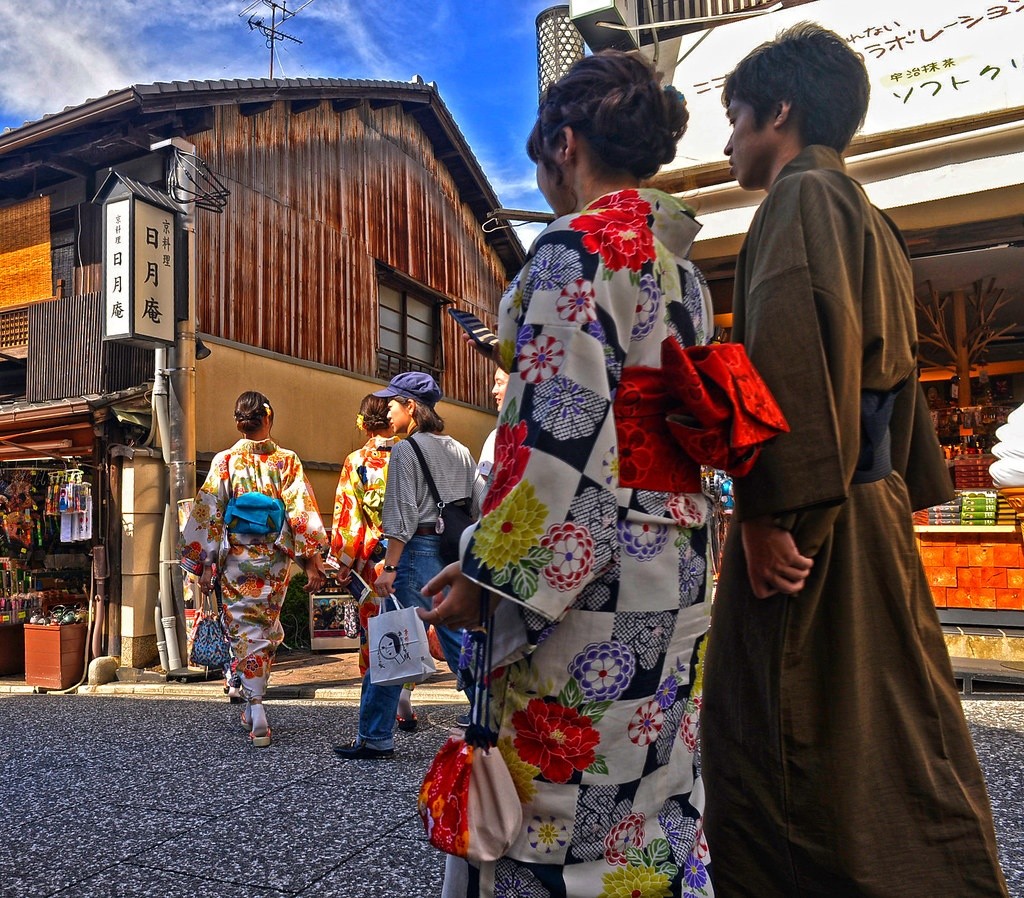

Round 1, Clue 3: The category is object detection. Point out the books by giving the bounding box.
[344,569,373,606]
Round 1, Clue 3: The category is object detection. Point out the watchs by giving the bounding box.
[383,564,398,573]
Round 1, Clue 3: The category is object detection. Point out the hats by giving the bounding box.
[372,371,441,407]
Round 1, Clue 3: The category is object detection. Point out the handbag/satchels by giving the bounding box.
[435,497,474,563]
[189,591,231,668]
[416,589,523,862]
[367,593,437,687]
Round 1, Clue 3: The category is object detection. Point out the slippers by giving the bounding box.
[241,713,253,730]
[250,728,272,746]
[397,711,417,731]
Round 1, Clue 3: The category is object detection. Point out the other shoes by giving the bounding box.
[334,739,394,759]
[224,686,229,694]
[230,694,247,704]
[456,714,471,727]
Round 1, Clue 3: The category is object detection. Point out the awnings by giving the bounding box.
[659,119,1024,259]
[0,422,95,459]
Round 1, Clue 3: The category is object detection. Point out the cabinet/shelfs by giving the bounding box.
[24,622,89,690]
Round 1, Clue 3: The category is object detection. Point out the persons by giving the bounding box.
[416,47,792,898]
[326,366,511,759]
[179,391,330,746]
[700,18,1009,898]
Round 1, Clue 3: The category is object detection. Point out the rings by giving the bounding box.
[204,587,207,589]
[209,589,212,592]
[381,587,386,592]
[433,607,440,617]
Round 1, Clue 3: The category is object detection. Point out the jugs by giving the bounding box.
[30,602,87,625]
